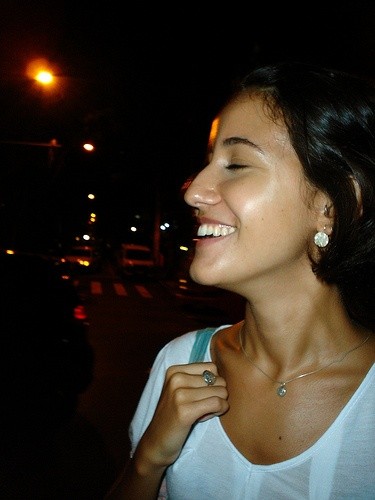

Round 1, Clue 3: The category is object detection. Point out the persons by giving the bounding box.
[105,66,375,500]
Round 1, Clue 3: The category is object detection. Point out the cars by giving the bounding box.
[116,242,157,280]
[60,243,101,271]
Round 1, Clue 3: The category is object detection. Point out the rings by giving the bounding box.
[202,370,216,386]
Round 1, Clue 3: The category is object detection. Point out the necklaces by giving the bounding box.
[239,321,373,397]
